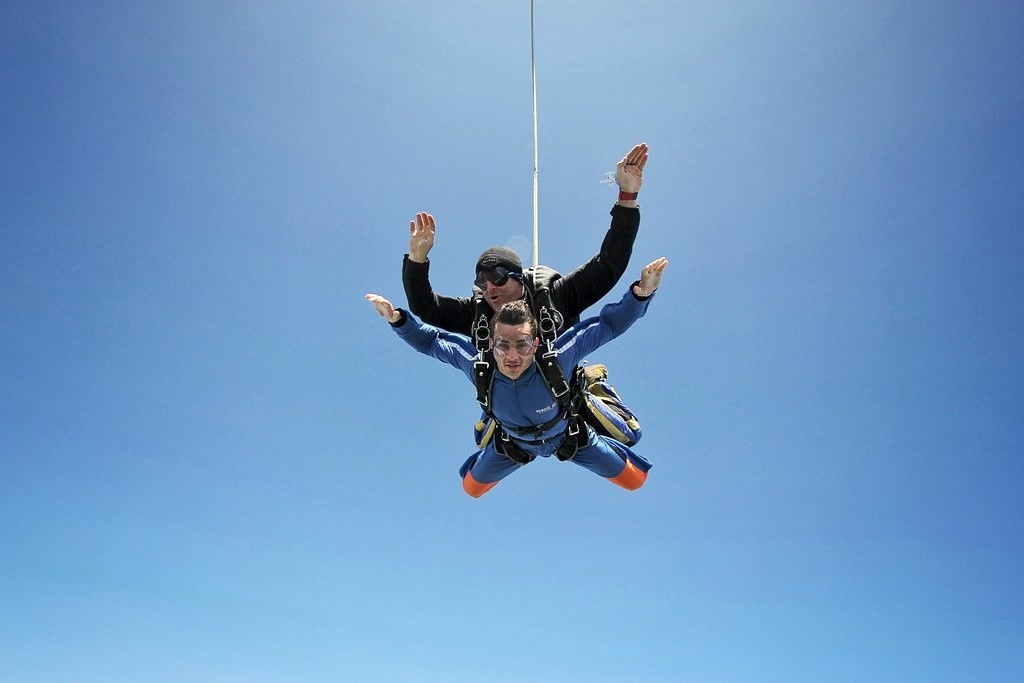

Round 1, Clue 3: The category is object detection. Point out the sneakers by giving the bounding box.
[585,364,608,382]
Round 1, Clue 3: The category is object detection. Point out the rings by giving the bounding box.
[626,163,631,165]
[654,267,658,271]
[631,164,635,165]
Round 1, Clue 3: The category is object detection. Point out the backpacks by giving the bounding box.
[470,265,561,338]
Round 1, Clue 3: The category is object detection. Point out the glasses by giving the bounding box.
[493,336,534,357]
[474,267,523,291]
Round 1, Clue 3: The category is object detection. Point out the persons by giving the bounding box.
[366,258,669,498]
[402,143,649,336]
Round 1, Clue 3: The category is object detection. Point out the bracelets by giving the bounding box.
[619,191,638,200]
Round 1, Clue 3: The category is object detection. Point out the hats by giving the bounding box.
[475,246,522,274]
